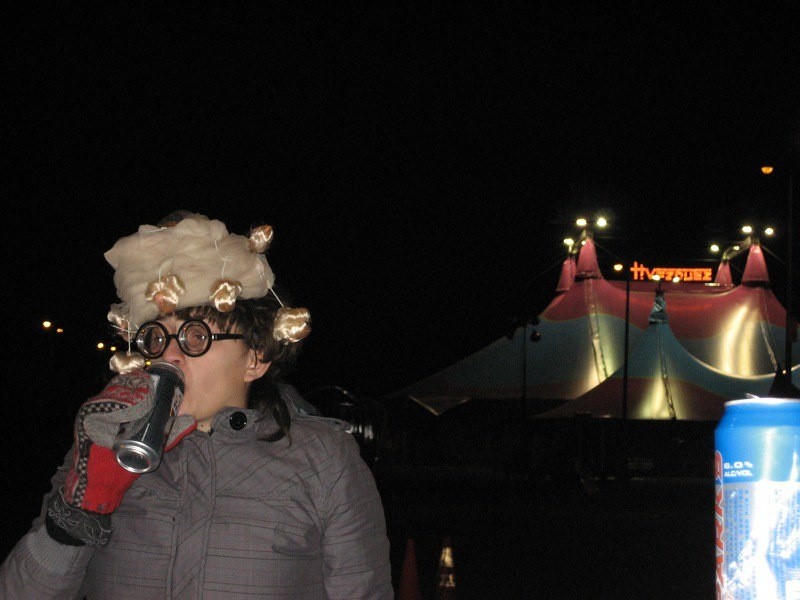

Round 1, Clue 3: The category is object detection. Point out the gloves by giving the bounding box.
[52,369,199,515]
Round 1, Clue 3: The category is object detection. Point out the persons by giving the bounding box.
[0,211,393,600]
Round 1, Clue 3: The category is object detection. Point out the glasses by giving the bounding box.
[133,319,252,359]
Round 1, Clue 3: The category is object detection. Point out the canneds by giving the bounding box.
[714,392,800,600]
[111,361,185,474]
